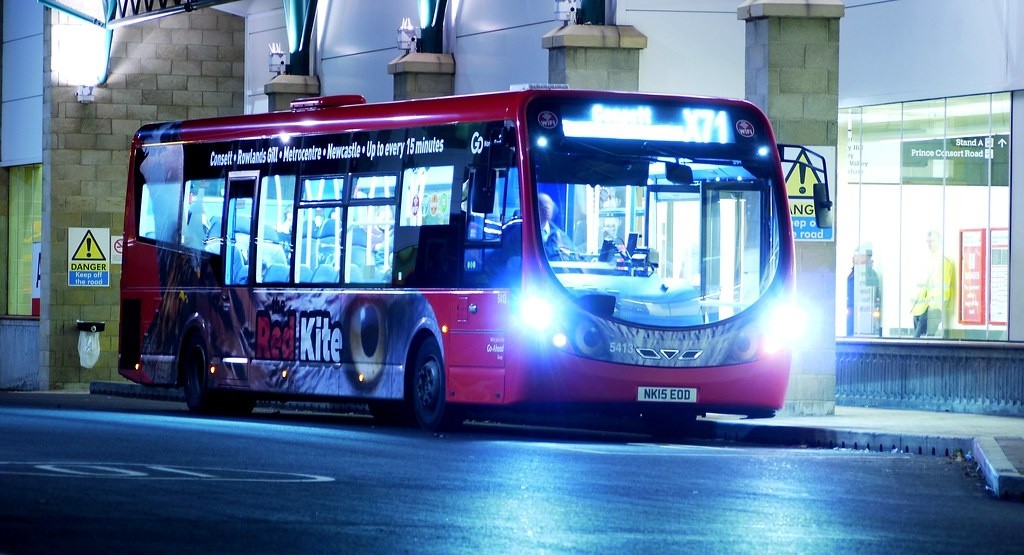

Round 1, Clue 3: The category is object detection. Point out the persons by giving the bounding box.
[280,200,394,261]
[910,231,956,337]
[538,192,580,275]
[494,209,522,275]
[847,244,882,337]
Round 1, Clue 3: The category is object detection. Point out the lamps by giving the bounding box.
[267,43,286,76]
[396,18,417,53]
[75,83,95,104]
[553,0,576,26]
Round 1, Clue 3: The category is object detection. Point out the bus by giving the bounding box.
[114,84,832,431]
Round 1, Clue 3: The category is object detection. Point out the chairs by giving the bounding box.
[202,215,366,284]
